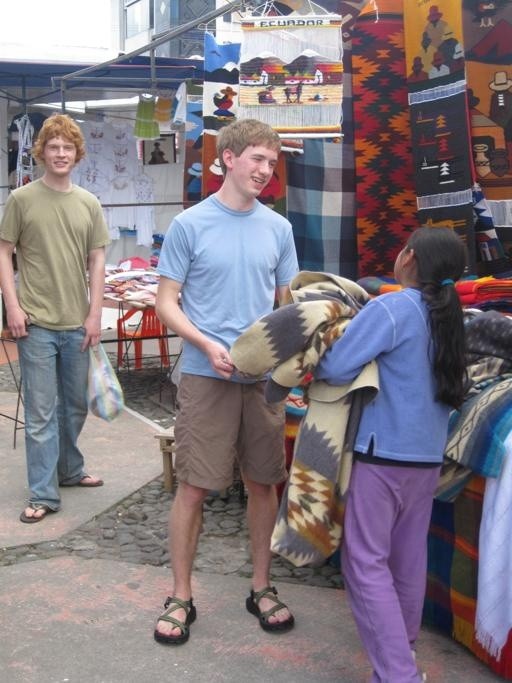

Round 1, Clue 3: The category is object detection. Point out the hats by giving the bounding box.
[220,86,237,96]
[467,88,480,109]
[489,71,512,91]
[411,4,464,71]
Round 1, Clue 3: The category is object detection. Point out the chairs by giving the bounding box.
[117,307,171,369]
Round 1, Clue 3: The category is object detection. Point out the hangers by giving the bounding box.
[197,0,285,33]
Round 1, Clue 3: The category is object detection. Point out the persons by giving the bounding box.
[312,225,473,683]
[151,116,300,645]
[0,111,114,523]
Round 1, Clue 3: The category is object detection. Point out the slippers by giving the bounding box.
[74,475,104,487]
[20,504,50,523]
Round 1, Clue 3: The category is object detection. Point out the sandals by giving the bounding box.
[154,596,196,645]
[246,587,295,634]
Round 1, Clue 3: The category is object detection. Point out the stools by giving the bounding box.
[154,425,229,499]
